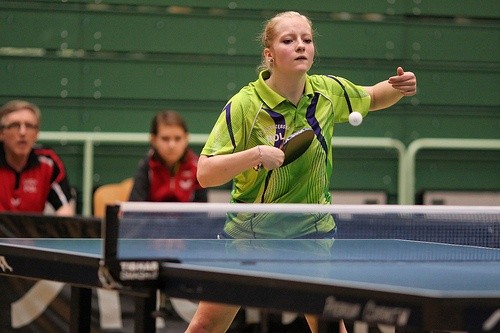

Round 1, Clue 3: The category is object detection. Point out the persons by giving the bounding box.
[128,109,209,203]
[0,99,76,218]
[183,11,416,333]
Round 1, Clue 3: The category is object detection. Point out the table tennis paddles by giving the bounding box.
[252,128,316,172]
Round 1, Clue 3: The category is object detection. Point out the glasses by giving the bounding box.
[0,122,38,131]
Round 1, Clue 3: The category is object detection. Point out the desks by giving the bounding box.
[0,201,500,333]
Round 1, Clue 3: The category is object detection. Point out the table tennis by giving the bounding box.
[347,111,363,127]
